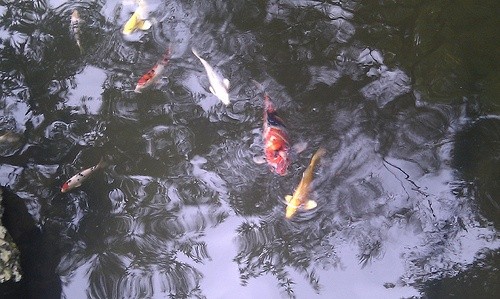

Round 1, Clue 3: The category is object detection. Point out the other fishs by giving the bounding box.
[70,9,86,55]
[120,0,152,35]
[0,130,21,143]
[250,78,308,177]
[60,156,108,193]
[191,47,231,107]
[124,45,173,94]
[284,147,326,219]
